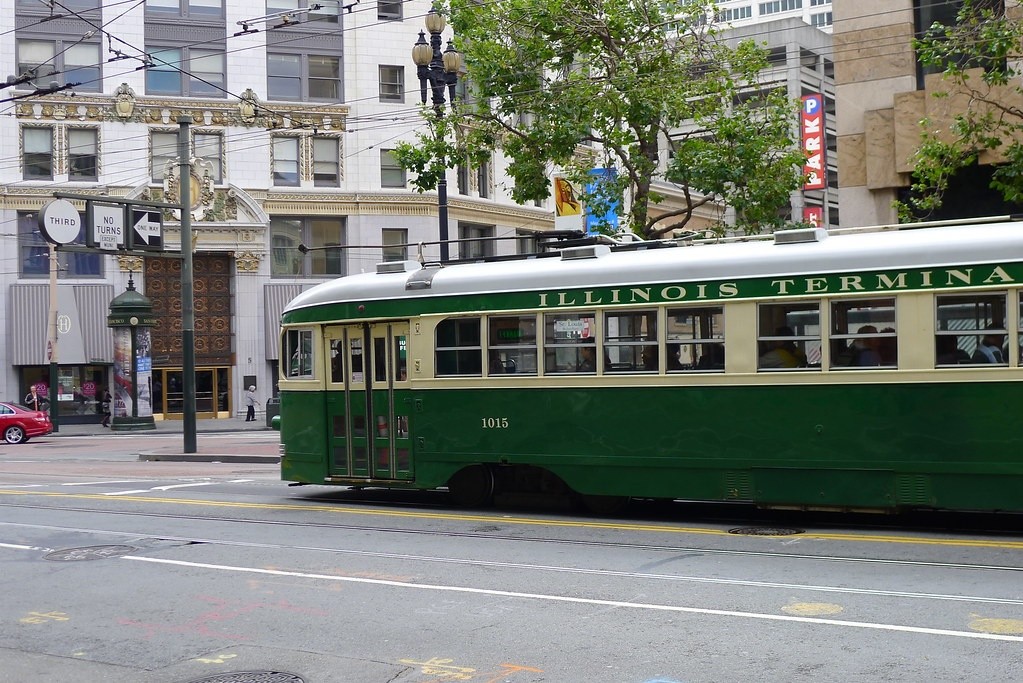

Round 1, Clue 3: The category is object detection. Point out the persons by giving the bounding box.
[934,333,971,364]
[641,347,649,363]
[400,371,406,381]
[834,325,880,369]
[665,339,684,370]
[825,330,848,359]
[25,386,42,411]
[245,385,260,421]
[972,323,1007,364]
[566,336,612,372]
[759,326,806,368]
[876,328,897,364]
[333,340,352,381]
[75,387,89,415]
[758,341,768,356]
[101,386,112,427]
[697,342,724,370]
[487,349,506,374]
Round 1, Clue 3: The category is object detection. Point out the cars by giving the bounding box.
[0,401,53,444]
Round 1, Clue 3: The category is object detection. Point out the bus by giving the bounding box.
[270,215,1023,518]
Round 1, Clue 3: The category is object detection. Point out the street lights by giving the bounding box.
[410,0,461,260]
